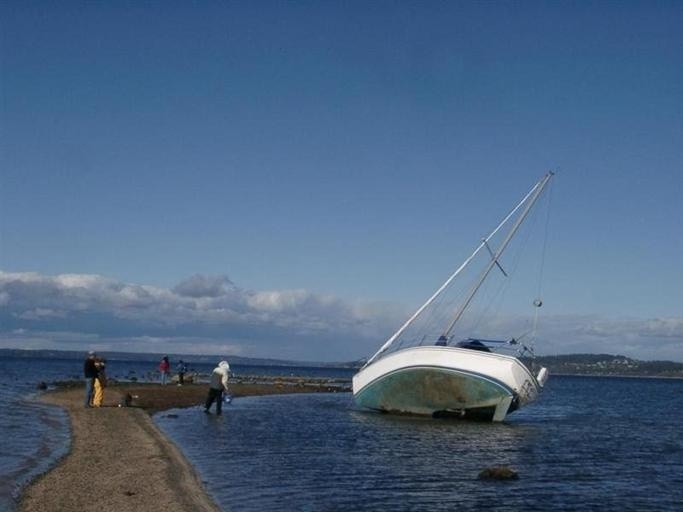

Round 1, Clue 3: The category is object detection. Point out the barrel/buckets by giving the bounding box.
[223,391,232,404]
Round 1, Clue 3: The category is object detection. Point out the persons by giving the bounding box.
[159,359,169,387]
[201,361,230,415]
[92,357,106,406]
[84,350,97,408]
[175,360,184,387]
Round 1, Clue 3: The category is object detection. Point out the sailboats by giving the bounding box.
[351,170,553,423]
